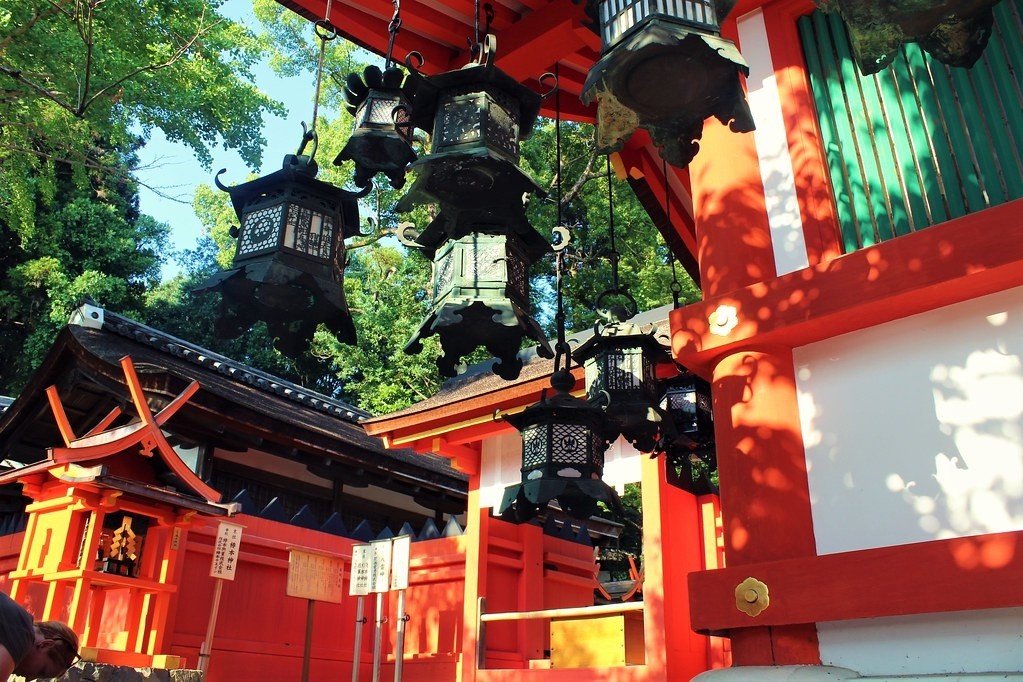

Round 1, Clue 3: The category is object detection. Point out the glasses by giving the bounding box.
[45,637,82,679]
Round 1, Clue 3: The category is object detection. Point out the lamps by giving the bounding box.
[192,0,375,359]
[390,0,547,218]
[78,510,150,579]
[499,61,629,522]
[572,156,670,452]
[396,204,557,382]
[332,0,420,188]
[653,157,718,474]
[579,0,756,168]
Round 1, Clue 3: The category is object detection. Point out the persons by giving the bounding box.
[0,591,81,682]
